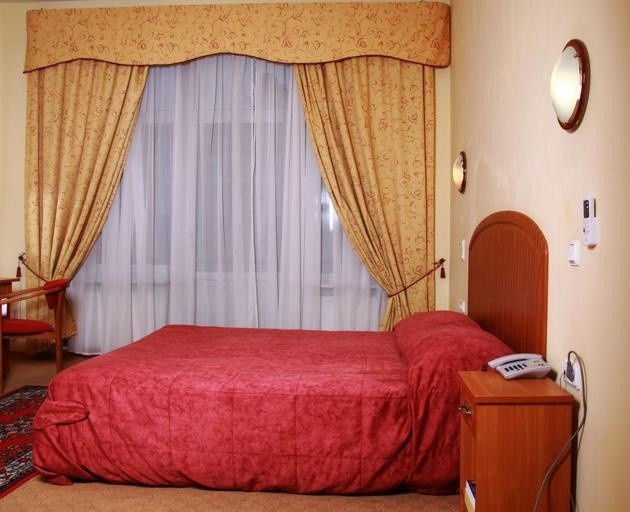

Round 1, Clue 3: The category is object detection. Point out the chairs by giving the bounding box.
[0,278,70,392]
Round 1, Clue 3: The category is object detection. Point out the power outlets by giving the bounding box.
[563,359,581,389]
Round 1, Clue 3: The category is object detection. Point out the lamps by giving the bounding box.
[453,151,467,193]
[551,40,591,134]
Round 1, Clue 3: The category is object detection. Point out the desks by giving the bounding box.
[0,278,20,317]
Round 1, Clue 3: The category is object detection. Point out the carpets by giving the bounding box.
[0,385,48,499]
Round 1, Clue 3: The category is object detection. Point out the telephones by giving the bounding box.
[488,353,551,378]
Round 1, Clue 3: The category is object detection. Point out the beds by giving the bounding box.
[33,210,549,494]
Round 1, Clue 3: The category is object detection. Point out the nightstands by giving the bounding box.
[458,370,573,512]
